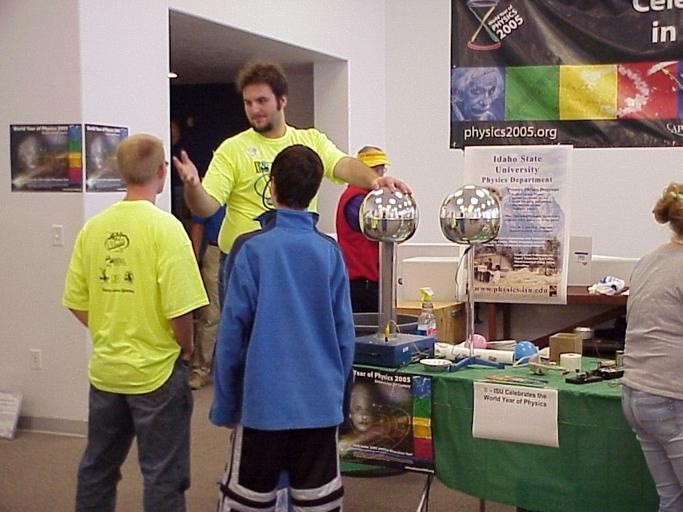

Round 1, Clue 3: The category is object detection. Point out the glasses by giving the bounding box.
[372,166,388,174]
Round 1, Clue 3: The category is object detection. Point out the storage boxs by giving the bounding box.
[393,300,467,345]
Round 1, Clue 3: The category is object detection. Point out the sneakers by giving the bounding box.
[188,365,214,390]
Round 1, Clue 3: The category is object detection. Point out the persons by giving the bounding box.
[336,145,392,313]
[187,150,226,389]
[362,209,403,236]
[619,182,682,511]
[86,131,111,173]
[444,210,465,241]
[345,382,387,448]
[15,134,64,189]
[209,144,356,512]
[451,66,505,122]
[171,59,411,315]
[483,257,500,283]
[61,133,211,512]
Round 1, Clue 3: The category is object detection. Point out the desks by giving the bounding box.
[474,286,629,360]
[337,356,659,512]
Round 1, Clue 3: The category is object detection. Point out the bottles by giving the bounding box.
[417,301,438,334]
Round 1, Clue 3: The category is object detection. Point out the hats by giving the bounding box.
[358,152,391,168]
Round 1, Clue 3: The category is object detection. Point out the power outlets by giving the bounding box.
[28,348,43,373]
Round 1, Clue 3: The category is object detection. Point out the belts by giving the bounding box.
[207,239,220,248]
[350,279,379,290]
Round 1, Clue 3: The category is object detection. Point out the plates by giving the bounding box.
[420,357,452,370]
